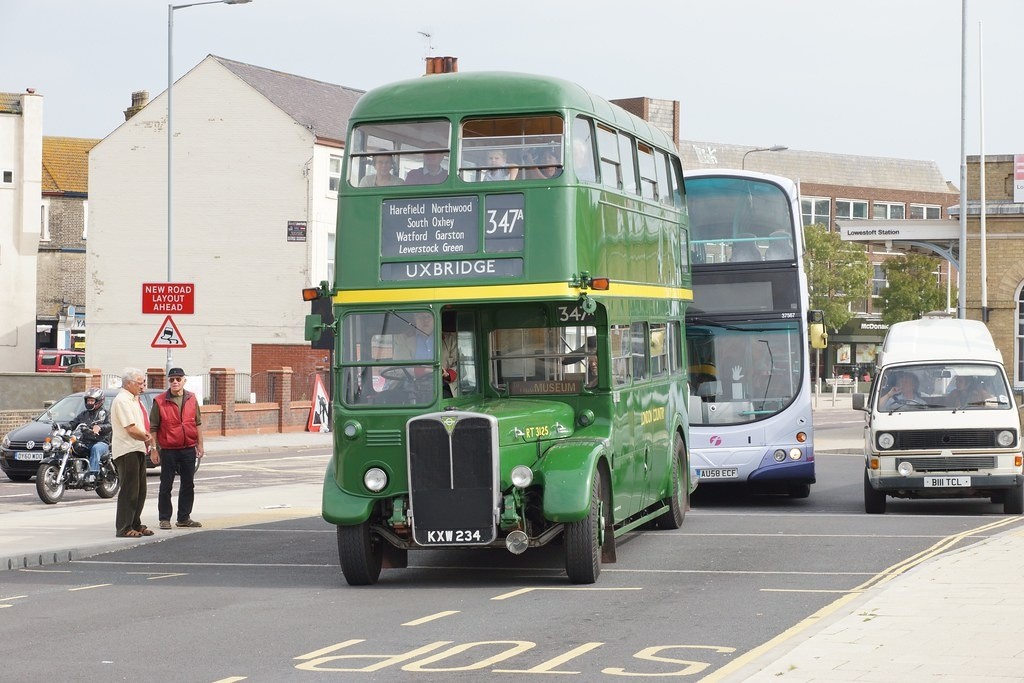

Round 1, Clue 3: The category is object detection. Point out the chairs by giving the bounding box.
[765,231,794,260]
[730,232,763,261]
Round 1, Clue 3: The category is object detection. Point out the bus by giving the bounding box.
[302,69,702,587]
[683,168,829,499]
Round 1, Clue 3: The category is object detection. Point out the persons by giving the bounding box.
[52,387,112,482]
[405,141,448,184]
[483,150,518,181]
[527,151,558,178]
[138,376,150,529]
[111,368,154,538]
[389,312,459,399]
[732,341,771,399]
[583,356,598,385]
[150,368,204,528]
[878,372,927,407]
[946,375,992,403]
[359,148,404,187]
[843,367,870,382]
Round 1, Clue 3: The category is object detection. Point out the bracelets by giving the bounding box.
[150,446,155,450]
[887,394,890,398]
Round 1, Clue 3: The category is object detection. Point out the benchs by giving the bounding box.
[825,378,855,393]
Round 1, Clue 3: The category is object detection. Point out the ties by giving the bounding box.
[138,397,150,454]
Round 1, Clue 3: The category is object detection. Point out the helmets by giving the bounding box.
[83,387,105,411]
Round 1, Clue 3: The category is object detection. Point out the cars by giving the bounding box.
[1,387,201,483]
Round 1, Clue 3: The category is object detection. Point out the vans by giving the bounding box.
[852,318,1023,515]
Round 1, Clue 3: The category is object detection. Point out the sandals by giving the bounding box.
[136,528,154,535]
[117,528,143,537]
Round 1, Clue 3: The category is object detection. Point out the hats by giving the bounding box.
[168,368,186,378]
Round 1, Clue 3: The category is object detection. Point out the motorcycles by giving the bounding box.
[36,410,120,505]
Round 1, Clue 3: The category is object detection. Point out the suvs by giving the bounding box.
[36,347,86,372]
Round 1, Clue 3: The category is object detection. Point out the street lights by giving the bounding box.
[164,0,253,393]
[741,144,788,170]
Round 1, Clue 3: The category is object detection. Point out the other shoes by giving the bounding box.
[89,474,95,483]
[175,518,202,526]
[158,520,172,528]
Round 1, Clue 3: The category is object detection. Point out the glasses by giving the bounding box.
[132,380,144,387]
[168,378,184,383]
